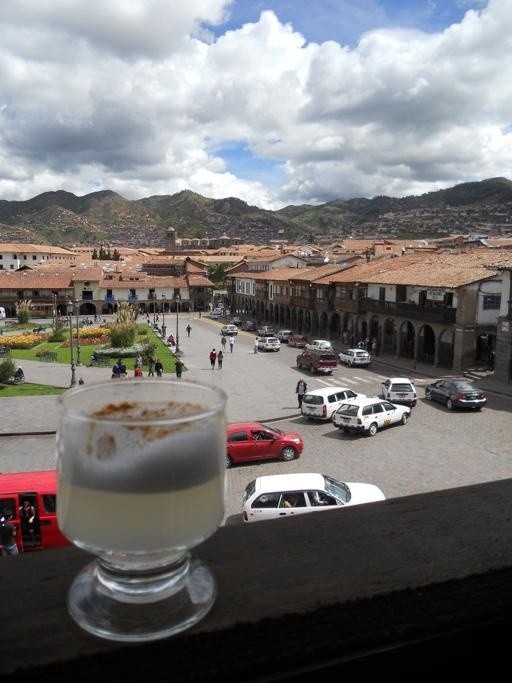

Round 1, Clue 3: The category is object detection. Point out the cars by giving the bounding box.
[241,469,388,521]
[231,316,242,325]
[275,330,291,341]
[425,377,488,414]
[304,339,332,350]
[332,397,411,434]
[0,306,6,320]
[289,333,307,347]
[339,348,370,367]
[210,291,225,319]
[224,419,305,468]
[242,320,257,331]
[382,376,418,405]
[258,325,274,336]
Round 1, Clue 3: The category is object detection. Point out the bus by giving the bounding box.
[16,301,58,320]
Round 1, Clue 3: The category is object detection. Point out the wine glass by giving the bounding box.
[49,374,230,645]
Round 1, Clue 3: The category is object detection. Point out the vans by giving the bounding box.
[299,385,367,421]
[0,470,79,552]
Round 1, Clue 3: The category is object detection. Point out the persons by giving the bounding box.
[221,335,226,352]
[209,349,216,369]
[343,330,377,355]
[174,359,184,377]
[0,510,20,558]
[255,337,258,353]
[296,378,308,408]
[112,353,164,378]
[186,324,191,336]
[217,351,223,368]
[229,335,234,352]
[19,501,36,547]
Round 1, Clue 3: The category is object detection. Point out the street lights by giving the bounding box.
[176,294,180,352]
[51,292,55,323]
[65,297,79,387]
[151,290,159,330]
[57,308,62,322]
[53,293,59,334]
[74,298,82,367]
[161,288,168,338]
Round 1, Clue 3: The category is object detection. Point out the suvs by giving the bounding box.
[258,335,282,353]
[221,324,237,335]
[297,348,337,375]
